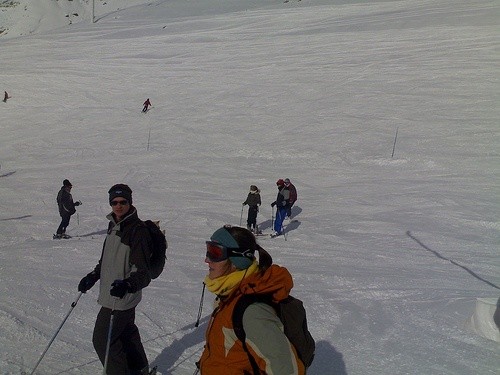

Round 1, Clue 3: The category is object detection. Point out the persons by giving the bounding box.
[76,184,152,374]
[141,98,151,112]
[197,223,306,374]
[284,178,297,219]
[55,179,81,237]
[269,179,290,237]
[242,184,262,233]
[3,91,8,102]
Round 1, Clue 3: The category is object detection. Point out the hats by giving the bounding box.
[248,185,257,191]
[276,179,290,185]
[63,179,71,187]
[108,184,131,202]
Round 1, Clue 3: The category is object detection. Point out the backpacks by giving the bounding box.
[132,220,167,280]
[231,290,316,375]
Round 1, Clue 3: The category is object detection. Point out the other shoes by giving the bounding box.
[246,230,257,235]
[270,230,280,238]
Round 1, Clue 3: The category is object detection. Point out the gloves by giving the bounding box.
[76,275,93,293]
[110,279,129,298]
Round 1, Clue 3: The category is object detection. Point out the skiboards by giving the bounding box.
[270,224,287,238]
[249,228,264,236]
[149,363,159,375]
[52,233,73,239]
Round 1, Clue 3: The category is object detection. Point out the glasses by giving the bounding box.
[205,241,244,262]
[111,201,127,205]
